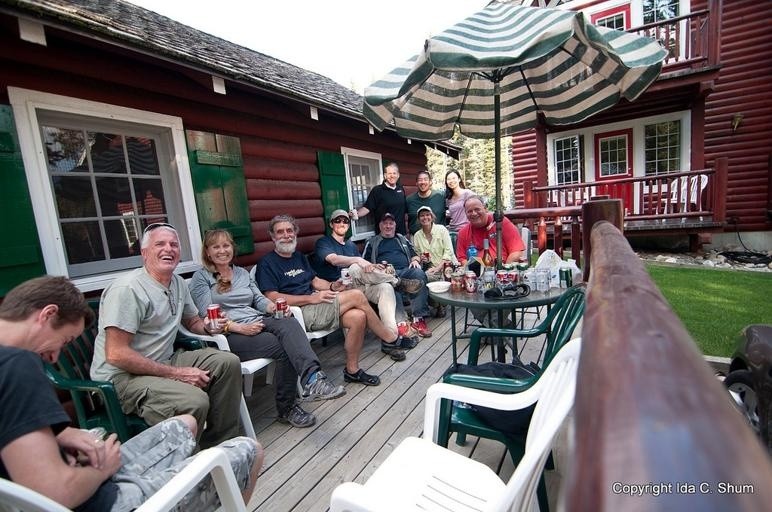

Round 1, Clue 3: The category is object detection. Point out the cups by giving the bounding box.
[466,244,477,260]
[482,272,494,291]
[523,267,551,294]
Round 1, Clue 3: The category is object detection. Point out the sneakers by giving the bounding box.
[398,277,424,294]
[302,371,347,402]
[381,307,446,362]
[343,367,380,386]
[277,404,316,428]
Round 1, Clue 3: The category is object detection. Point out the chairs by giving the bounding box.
[656,174,709,221]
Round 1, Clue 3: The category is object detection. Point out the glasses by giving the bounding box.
[145,223,176,232]
[168,293,177,317]
[332,218,349,224]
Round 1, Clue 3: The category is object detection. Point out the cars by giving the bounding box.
[716,324,770,464]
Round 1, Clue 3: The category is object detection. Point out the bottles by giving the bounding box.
[483,239,495,271]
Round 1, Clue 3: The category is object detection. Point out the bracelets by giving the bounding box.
[223,321,232,334]
[329,283,334,291]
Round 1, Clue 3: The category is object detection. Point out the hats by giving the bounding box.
[382,213,395,221]
[331,209,350,219]
[417,205,431,214]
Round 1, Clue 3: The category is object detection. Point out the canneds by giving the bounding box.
[381,260,388,269]
[523,267,551,292]
[496,269,520,286]
[90,427,108,440]
[275,297,288,321]
[444,260,453,282]
[451,271,476,293]
[351,209,359,221]
[207,303,221,334]
[341,268,352,287]
[559,267,573,289]
[423,252,430,263]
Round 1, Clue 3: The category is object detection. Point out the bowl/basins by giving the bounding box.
[426,281,451,293]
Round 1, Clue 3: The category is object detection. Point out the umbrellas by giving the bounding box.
[362,0,670,270]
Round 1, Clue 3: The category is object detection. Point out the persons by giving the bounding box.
[349,162,527,344]
[0,275,265,511]
[188,230,346,428]
[90,223,242,456]
[256,214,416,387]
[314,209,406,361]
